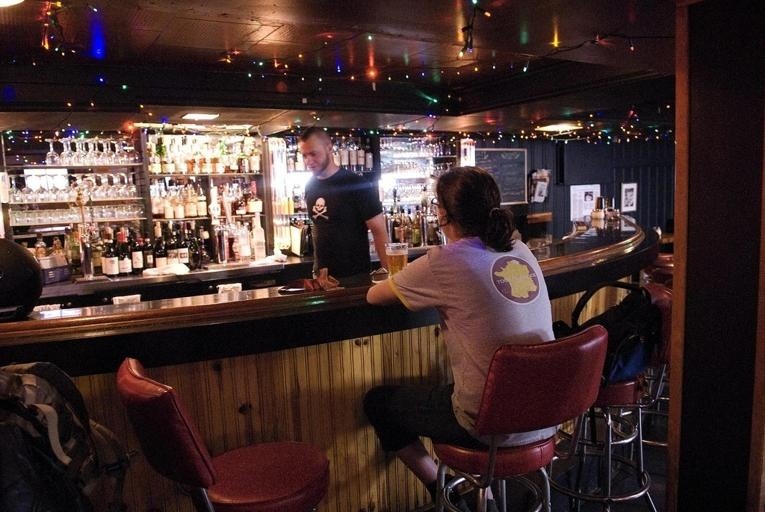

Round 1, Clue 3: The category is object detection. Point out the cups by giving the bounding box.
[385,242,408,277]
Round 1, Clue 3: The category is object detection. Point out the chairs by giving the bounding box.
[433,324,608,512]
[115,356,329,512]
[547,282,674,512]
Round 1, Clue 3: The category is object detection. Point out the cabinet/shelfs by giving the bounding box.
[144,123,270,261]
[380,130,459,250]
[265,126,380,260]
[2,127,151,279]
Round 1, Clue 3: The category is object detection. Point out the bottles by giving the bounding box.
[35,232,46,259]
[382,204,423,248]
[287,136,374,173]
[218,179,261,216]
[215,214,263,263]
[101,217,201,279]
[150,137,263,175]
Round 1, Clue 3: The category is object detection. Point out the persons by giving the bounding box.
[297,125,391,279]
[363,166,563,512]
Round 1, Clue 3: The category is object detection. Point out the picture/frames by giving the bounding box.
[621,183,637,213]
[569,184,601,222]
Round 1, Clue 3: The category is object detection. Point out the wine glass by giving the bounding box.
[7,173,139,201]
[45,137,141,164]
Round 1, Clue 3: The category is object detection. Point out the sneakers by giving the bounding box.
[433,494,470,512]
[487,500,498,512]
